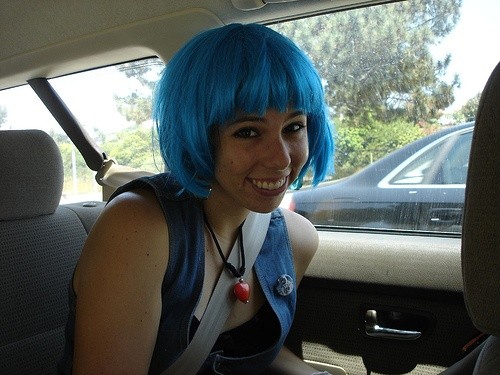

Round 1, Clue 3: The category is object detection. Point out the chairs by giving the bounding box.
[432,142,468,184]
[1,129,109,375]
[462,59,500,375]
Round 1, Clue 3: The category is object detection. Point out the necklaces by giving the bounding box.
[202,206,250,306]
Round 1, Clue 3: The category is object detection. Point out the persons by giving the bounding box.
[57,22,338,374]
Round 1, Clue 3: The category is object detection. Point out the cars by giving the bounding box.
[288,120,477,239]
